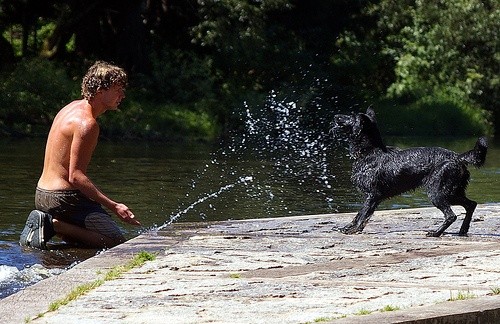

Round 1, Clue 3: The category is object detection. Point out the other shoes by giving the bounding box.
[30,210,56,252]
[19,210,42,248]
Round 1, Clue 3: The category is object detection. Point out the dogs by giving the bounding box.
[333,104,488,237]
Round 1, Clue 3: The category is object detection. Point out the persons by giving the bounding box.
[19,60,142,249]
